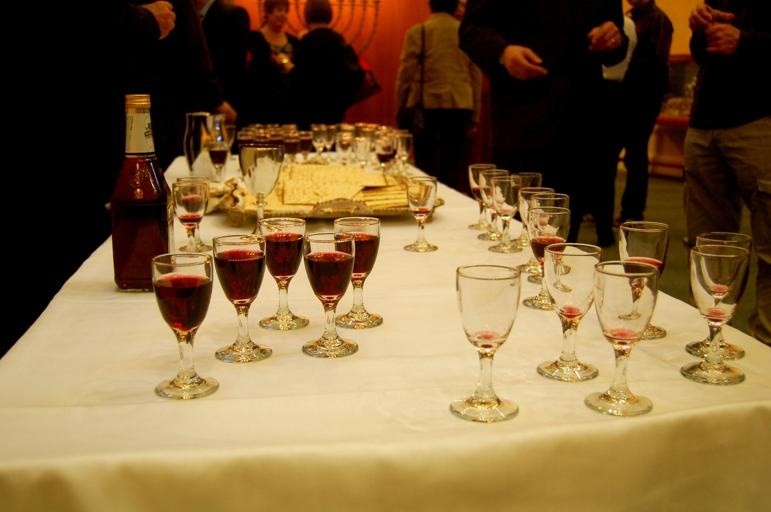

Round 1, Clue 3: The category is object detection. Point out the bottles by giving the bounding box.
[618,221,670,340]
[109,94,168,292]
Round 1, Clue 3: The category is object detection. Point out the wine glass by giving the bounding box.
[403,176,439,253]
[466,163,573,309]
[680,231,754,387]
[449,264,522,423]
[330,216,384,329]
[302,231,359,358]
[172,176,212,253]
[151,253,220,401]
[212,234,273,364]
[537,242,603,383]
[257,217,310,331]
[209,123,412,187]
[584,259,659,418]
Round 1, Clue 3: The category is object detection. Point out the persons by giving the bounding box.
[683,0,771,346]
[1,1,365,354]
[397,1,675,248]
